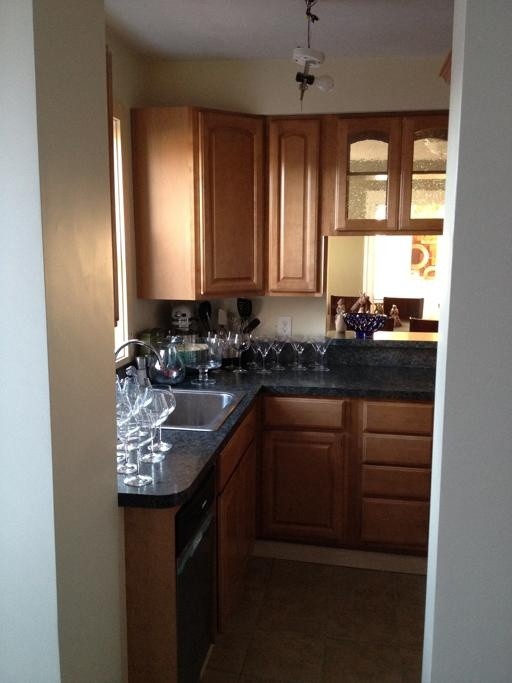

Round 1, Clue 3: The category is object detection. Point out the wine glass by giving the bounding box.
[184,332,334,387]
[116,367,177,486]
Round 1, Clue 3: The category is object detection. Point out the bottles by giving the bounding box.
[135,356,154,382]
[148,335,185,385]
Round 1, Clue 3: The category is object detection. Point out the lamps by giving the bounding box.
[291,0,336,104]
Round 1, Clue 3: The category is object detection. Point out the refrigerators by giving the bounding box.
[174,460,218,680]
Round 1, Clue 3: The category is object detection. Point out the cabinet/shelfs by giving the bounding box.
[260,395,435,551]
[124,462,216,681]
[131,105,328,301]
[320,110,447,236]
[216,401,261,633]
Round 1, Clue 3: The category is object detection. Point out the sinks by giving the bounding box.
[158,388,247,433]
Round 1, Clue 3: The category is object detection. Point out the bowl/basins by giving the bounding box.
[344,313,388,339]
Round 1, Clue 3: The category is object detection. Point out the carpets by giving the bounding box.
[238,556,427,683]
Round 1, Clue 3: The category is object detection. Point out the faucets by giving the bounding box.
[114,338,164,370]
